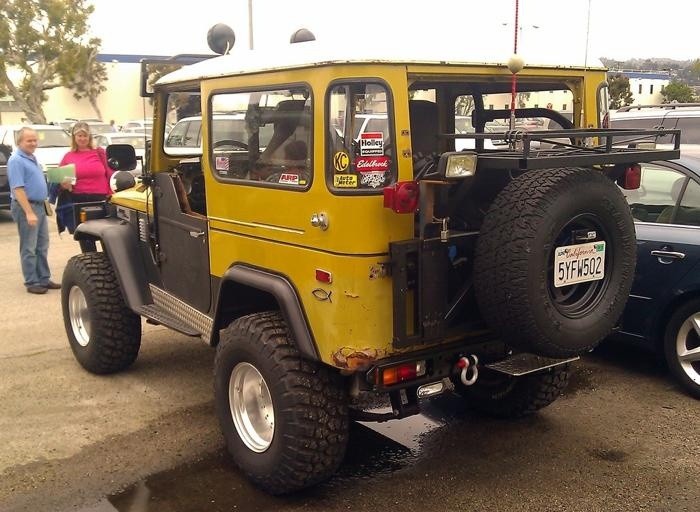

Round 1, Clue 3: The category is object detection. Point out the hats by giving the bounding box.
[73,123,90,135]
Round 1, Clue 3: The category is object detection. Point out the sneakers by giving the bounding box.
[27,286,48,293]
[45,281,61,289]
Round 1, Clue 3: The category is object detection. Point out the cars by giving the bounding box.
[1,118,177,210]
[601,157,699,398]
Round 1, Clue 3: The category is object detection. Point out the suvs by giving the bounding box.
[60,23,681,497]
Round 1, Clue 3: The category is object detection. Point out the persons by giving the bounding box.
[56,121,119,254]
[5,126,62,296]
[263,139,312,187]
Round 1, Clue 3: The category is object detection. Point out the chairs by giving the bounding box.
[656,176,688,223]
[409,99,446,150]
[252,99,311,180]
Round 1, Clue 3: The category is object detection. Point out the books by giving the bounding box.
[46,163,77,185]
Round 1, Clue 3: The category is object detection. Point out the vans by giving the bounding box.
[567,104,700,157]
[353,114,498,153]
[164,113,344,166]
